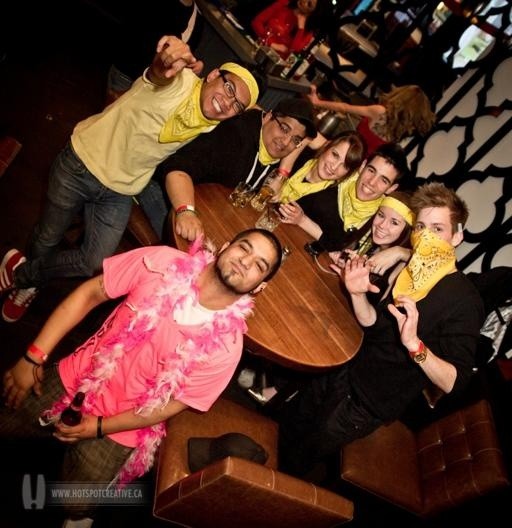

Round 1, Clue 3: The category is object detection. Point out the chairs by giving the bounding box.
[322,396,509,519]
[143,390,354,528]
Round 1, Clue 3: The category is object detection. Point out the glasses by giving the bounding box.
[220,71,245,117]
[274,115,302,145]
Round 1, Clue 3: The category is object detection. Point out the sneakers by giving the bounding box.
[0,249,39,322]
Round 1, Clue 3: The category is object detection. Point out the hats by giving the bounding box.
[188,432,269,473]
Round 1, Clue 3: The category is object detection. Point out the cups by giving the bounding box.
[254,206,281,233]
[227,180,256,209]
[250,167,290,212]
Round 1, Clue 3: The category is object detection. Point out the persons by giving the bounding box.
[2,229,282,528]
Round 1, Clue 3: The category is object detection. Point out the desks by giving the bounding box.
[166,178,367,396]
[306,23,384,107]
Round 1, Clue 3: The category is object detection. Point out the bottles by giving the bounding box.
[280,38,325,81]
[60,392,87,425]
